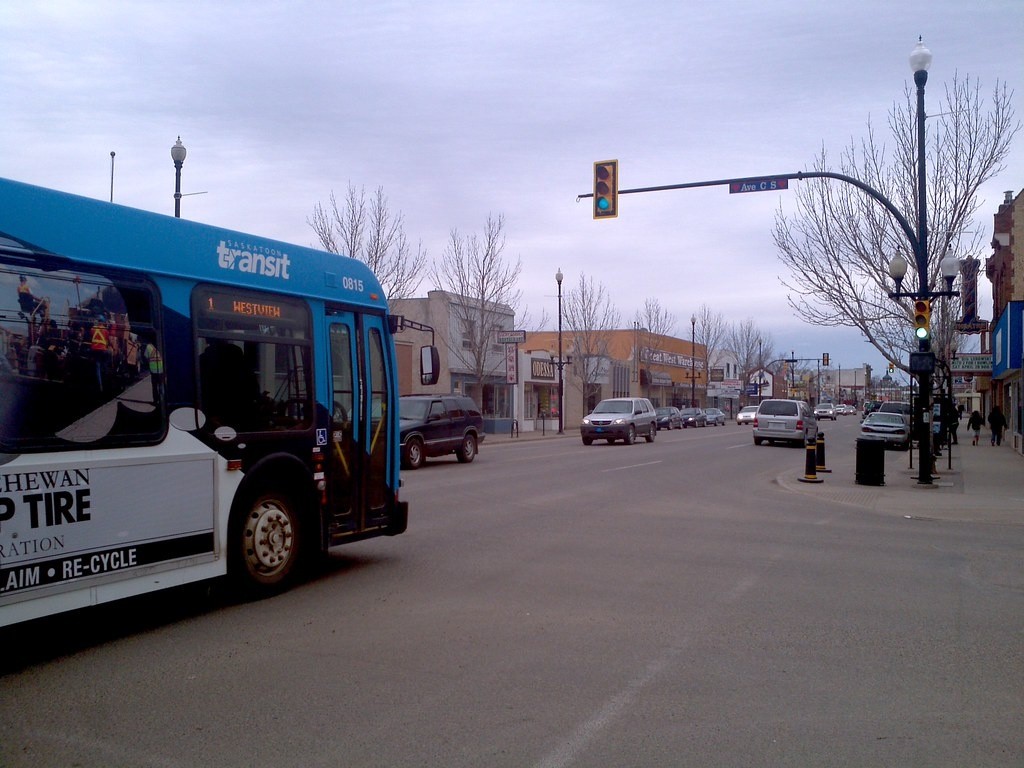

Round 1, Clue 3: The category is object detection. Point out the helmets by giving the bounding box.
[97,314,105,322]
[19,275,28,281]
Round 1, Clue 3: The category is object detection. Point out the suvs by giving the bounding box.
[752,399,818,446]
[348,394,483,462]
[581,398,657,445]
[878,401,916,426]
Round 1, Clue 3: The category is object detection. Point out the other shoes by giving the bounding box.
[951,442,958,444]
[992,441,994,446]
[972,440,975,446]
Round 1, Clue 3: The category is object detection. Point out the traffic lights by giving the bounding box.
[592,159,619,220]
[913,297,930,343]
[889,362,894,373]
[823,352,829,366]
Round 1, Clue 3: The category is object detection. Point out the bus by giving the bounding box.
[0,179,441,624]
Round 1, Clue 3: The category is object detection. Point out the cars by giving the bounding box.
[815,402,856,420]
[736,405,758,426]
[860,410,910,446]
[655,402,726,433]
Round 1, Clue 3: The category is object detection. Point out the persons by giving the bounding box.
[930,399,945,456]
[6,275,162,402]
[988,406,1008,446]
[967,411,986,446]
[949,404,961,443]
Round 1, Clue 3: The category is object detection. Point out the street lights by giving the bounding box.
[109,151,115,203]
[548,268,574,435]
[755,338,762,407]
[887,35,964,485]
[691,313,697,408]
[171,136,186,216]
[789,349,797,389]
